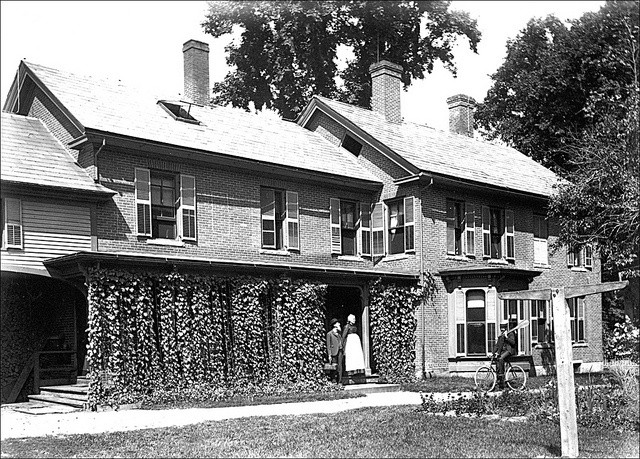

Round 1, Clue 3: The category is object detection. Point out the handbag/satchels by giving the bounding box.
[324,360,338,375]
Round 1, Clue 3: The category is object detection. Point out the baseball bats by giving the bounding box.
[504,319,528,334]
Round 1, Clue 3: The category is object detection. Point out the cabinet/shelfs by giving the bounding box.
[33,349,77,395]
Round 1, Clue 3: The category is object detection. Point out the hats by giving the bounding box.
[329,318,341,326]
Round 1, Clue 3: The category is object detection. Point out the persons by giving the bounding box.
[342,314,365,384]
[326,317,343,384]
[488,326,517,391]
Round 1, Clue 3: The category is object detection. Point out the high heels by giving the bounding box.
[349,379,355,384]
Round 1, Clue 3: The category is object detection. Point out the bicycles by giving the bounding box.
[473,355,526,391]
